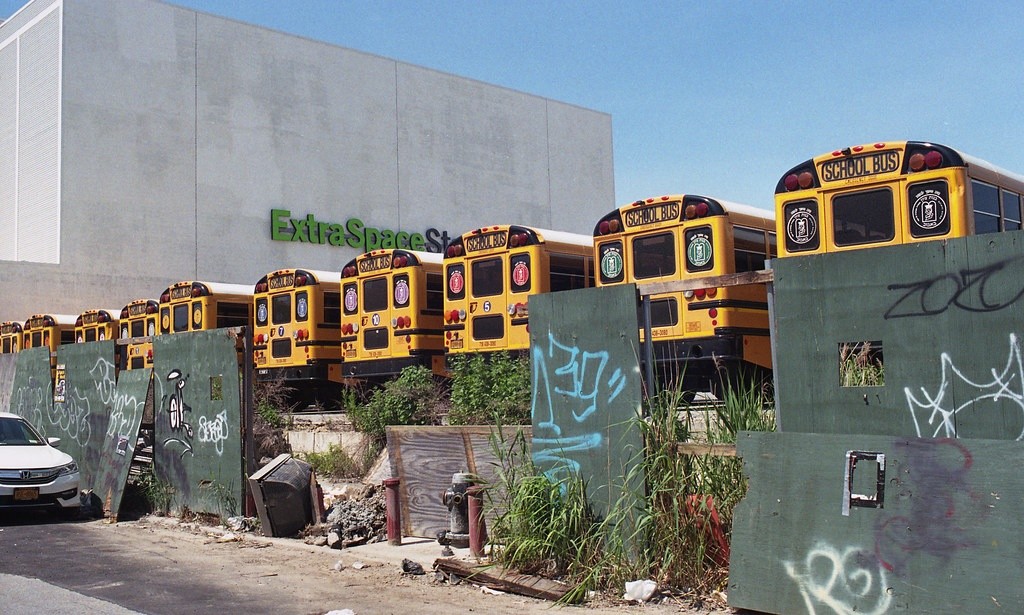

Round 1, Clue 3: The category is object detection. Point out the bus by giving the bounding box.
[339,249,444,403]
[253,268,348,413]
[774,140,1023,354]
[117,298,161,384]
[592,194,775,409]
[0,321,27,354]
[158,281,255,366]
[23,313,80,389]
[443,225,594,368]
[74,308,122,377]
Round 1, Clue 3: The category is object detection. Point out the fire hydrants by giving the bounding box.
[443,471,480,549]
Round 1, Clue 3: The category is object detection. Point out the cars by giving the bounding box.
[0,411,81,521]
